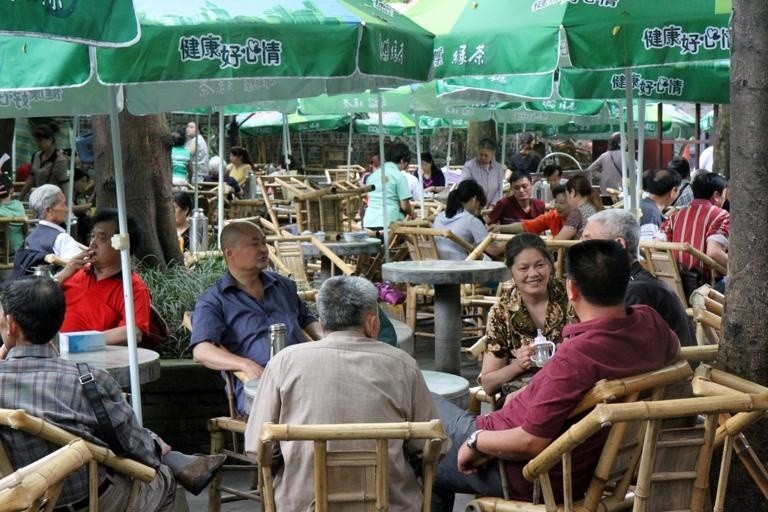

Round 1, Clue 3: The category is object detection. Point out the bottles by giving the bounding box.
[530,329,555,368]
[269,323,288,358]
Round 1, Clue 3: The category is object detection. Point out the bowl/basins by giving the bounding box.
[343,231,368,242]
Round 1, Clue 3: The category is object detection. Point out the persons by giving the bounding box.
[71,169,94,216]
[171,131,194,191]
[659,172,731,295]
[553,173,607,272]
[172,191,193,252]
[227,146,255,192]
[430,178,507,261]
[487,184,572,236]
[582,208,697,430]
[0,174,29,255]
[23,183,88,263]
[431,238,682,512]
[504,131,543,181]
[483,171,546,234]
[640,168,682,226]
[18,123,70,202]
[243,275,453,512]
[587,131,638,196]
[479,233,578,410]
[413,151,445,193]
[360,152,380,204]
[667,155,695,208]
[531,164,569,202]
[0,274,228,512]
[189,220,323,416]
[183,122,210,191]
[203,155,245,198]
[401,164,420,200]
[361,142,415,245]
[457,138,504,210]
[52,210,152,346]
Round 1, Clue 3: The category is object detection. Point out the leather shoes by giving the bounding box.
[177,452,230,496]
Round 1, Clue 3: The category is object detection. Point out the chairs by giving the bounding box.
[184,312,291,512]
[259,234,354,320]
[224,217,280,275]
[495,178,633,286]
[466,363,691,512]
[188,169,290,220]
[0,217,31,274]
[691,284,726,329]
[390,223,492,261]
[695,363,767,512]
[260,173,376,236]
[638,241,727,309]
[381,157,498,230]
[0,408,156,512]
[257,419,448,512]
[521,395,767,511]
[11,251,74,282]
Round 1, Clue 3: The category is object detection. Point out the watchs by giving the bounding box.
[466,428,485,455]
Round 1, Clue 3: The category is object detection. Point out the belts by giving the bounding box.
[53,466,117,512]
